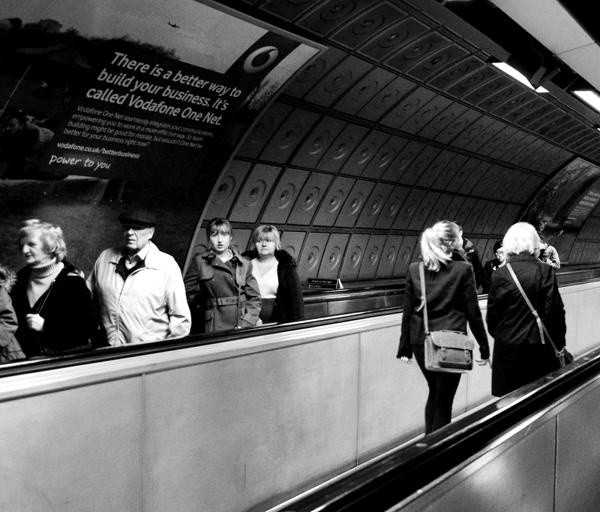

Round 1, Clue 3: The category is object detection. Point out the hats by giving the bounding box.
[119,208,155,224]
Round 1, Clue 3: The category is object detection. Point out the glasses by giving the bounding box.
[123,223,152,230]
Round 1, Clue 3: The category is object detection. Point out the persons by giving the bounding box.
[0,262,26,363]
[538,242,562,271]
[78,207,192,346]
[5,218,94,362]
[182,215,262,338]
[483,221,567,397]
[395,218,490,433]
[450,221,482,291]
[483,240,505,294]
[237,221,303,328]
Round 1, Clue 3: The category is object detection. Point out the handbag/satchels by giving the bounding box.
[558,346,573,367]
[425,330,473,374]
[39,345,92,357]
[235,314,263,329]
